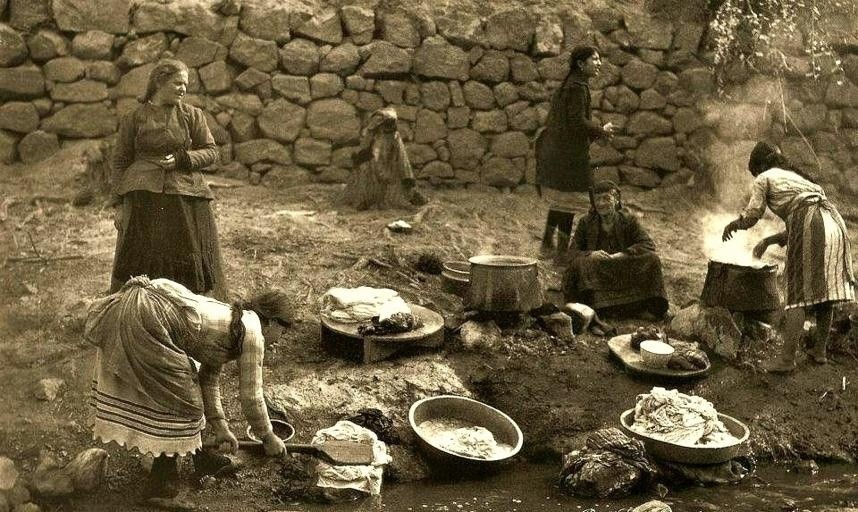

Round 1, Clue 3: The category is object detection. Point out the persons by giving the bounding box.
[534,44,614,263]
[723,142,856,374]
[338,108,430,211]
[82,273,296,510]
[563,180,669,315]
[109,58,219,294]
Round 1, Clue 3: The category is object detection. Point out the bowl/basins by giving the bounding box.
[246,419,296,443]
[641,338,675,369]
[441,259,470,295]
[409,395,524,470]
[620,408,749,463]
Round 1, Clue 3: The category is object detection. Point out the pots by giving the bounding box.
[465,255,543,314]
[700,259,782,312]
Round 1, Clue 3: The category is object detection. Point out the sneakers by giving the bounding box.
[765,357,796,373]
[804,346,828,365]
